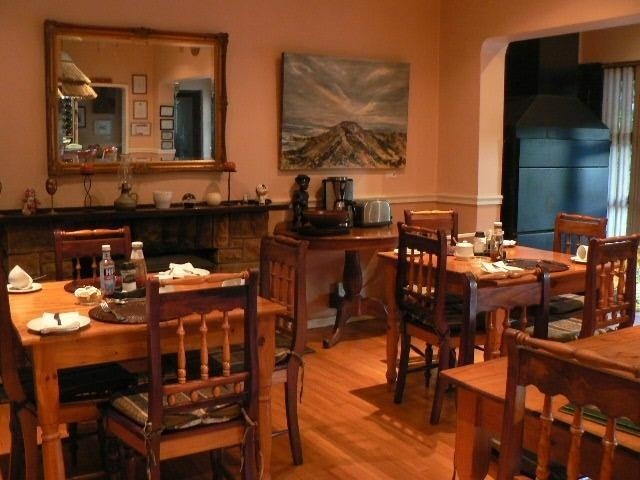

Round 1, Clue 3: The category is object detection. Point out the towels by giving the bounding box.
[578,242,591,261]
[40,310,81,334]
[7,263,35,293]
[169,260,201,281]
[502,236,516,245]
[394,246,423,256]
[480,260,524,276]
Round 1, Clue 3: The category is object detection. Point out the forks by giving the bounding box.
[54,313,61,325]
[101,301,124,320]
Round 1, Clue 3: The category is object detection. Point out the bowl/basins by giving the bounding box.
[304,207,349,228]
[482,266,523,273]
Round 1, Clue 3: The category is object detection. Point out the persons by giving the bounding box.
[291,174,310,232]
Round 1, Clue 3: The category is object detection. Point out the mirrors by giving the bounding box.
[44,18,229,174]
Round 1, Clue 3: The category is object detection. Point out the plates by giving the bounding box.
[571,256,587,263]
[27,316,91,334]
[7,283,42,293]
[164,267,210,276]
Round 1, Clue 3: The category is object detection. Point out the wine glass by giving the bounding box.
[46,178,59,215]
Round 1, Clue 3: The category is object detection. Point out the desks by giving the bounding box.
[275,221,399,348]
[439,325,640,480]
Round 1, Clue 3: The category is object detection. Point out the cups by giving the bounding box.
[455,240,472,257]
[470,256,490,268]
[153,191,173,207]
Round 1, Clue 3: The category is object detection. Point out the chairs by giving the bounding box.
[1,272,139,479]
[497,328,629,479]
[393,221,487,426]
[550,212,608,314]
[511,231,640,343]
[102,270,262,479]
[463,263,551,478]
[403,209,459,247]
[202,233,310,465]
[53,225,132,282]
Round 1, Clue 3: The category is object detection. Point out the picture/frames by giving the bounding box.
[160,106,174,118]
[131,122,151,136]
[160,119,175,131]
[132,74,148,95]
[94,120,112,135]
[77,106,86,129]
[279,50,412,170]
[161,141,173,151]
[133,100,148,120]
[162,131,173,141]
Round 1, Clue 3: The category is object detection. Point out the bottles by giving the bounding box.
[120,261,137,291]
[100,244,115,297]
[490,222,504,261]
[131,242,148,288]
[473,232,486,254]
